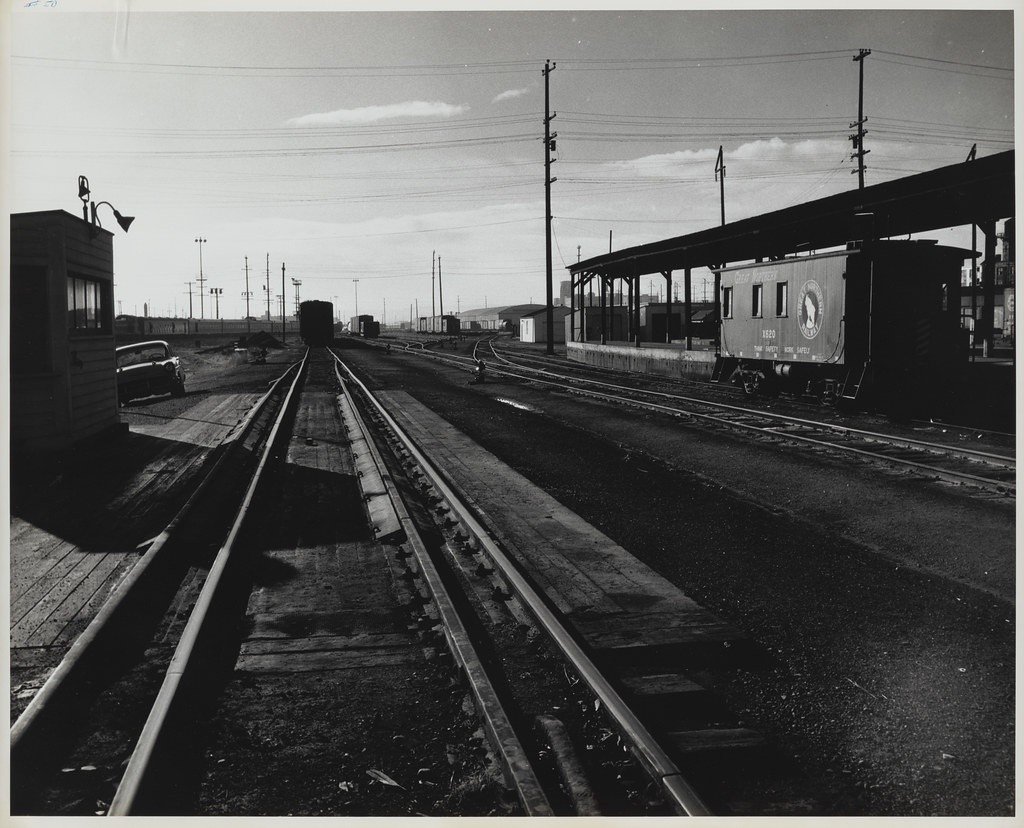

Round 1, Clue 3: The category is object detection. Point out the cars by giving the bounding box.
[114,342,187,407]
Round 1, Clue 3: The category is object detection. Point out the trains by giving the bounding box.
[349,315,381,340]
[715,241,974,418]
[298,300,335,349]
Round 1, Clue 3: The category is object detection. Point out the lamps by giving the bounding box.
[92,201,135,239]
